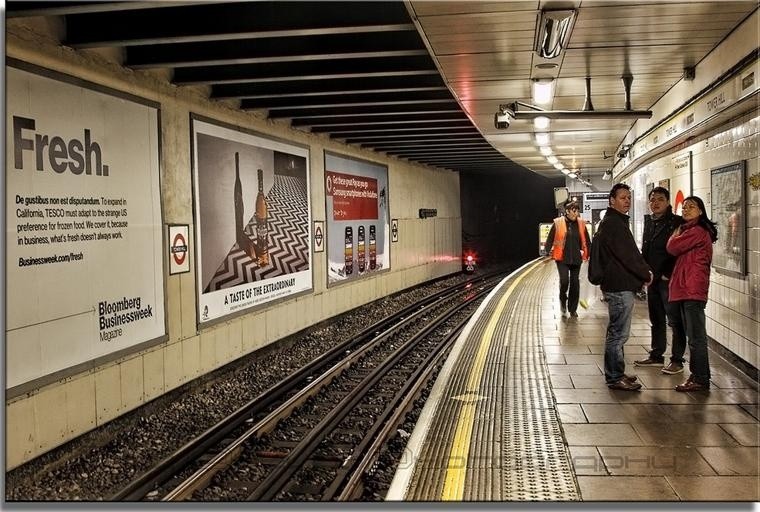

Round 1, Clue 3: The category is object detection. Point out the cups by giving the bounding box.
[580,299,589,309]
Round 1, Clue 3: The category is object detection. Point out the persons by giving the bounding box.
[587,182,654,392]
[634,186,688,375]
[544,199,592,319]
[665,196,719,393]
[724,199,742,274]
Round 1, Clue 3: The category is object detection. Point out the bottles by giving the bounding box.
[240,230,257,261]
[636,285,648,302]
[253,170,270,265]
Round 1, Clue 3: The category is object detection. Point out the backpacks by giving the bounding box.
[588,232,604,284]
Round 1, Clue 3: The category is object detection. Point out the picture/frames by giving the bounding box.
[710,159,746,280]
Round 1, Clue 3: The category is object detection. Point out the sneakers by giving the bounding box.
[609,360,710,390]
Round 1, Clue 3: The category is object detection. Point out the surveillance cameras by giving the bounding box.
[618,150,626,158]
[495,113,509,129]
[601,171,612,181]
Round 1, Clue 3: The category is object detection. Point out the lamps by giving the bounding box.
[495,77,632,186]
